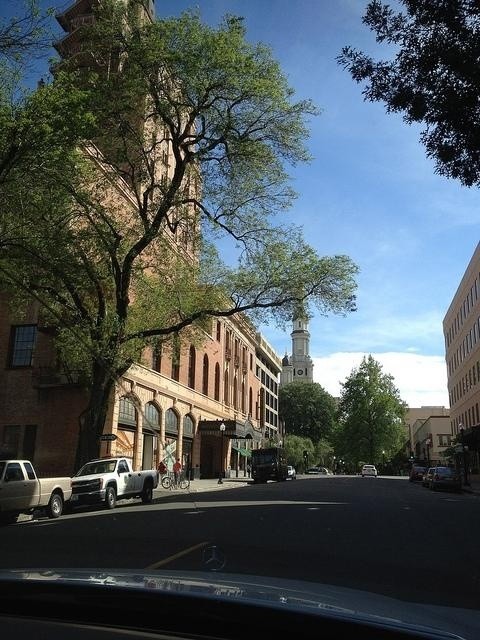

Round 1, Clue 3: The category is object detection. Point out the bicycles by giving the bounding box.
[161,469,190,489]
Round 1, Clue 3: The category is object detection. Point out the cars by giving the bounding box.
[307,467,328,475]
[362,465,378,478]
[0,545,480,640]
[410,466,462,492]
[287,466,296,481]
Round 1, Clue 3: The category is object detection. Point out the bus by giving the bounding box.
[251,447,288,484]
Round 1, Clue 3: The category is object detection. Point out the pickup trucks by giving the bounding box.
[71,457,159,509]
[0,459,73,524]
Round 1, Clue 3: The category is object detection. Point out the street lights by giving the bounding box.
[382,450,385,475]
[458,422,469,486]
[279,440,283,447]
[304,451,308,474]
[426,439,431,466]
[333,456,345,475]
[218,423,226,484]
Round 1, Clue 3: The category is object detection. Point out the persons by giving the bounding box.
[157,461,169,486]
[173,458,182,487]
